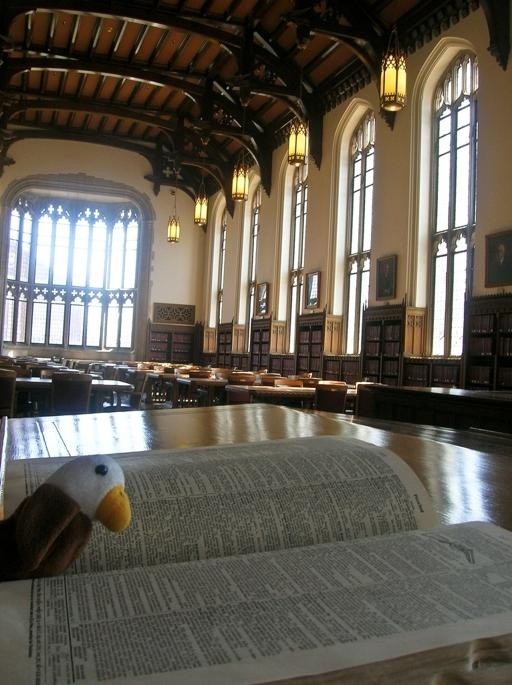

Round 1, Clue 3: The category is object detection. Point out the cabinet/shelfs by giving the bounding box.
[324,356,360,381]
[251,320,270,370]
[464,296,512,390]
[217,324,231,366]
[147,319,202,361]
[297,312,324,378]
[364,306,402,383]
[270,355,296,374]
[232,353,250,369]
[202,355,217,366]
[402,358,463,389]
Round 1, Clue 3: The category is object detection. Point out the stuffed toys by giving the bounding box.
[0,454,132,582]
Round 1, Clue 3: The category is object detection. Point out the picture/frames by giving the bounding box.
[484,229,512,289]
[304,271,321,310]
[255,281,269,316]
[375,253,398,301]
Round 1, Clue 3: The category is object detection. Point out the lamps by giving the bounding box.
[167,171,181,246]
[288,113,308,168]
[194,169,208,228]
[379,21,407,112]
[231,144,251,204]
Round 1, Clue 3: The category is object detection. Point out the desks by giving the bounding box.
[356,380,512,437]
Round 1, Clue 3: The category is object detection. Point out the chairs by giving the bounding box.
[54,357,356,412]
[1,353,133,417]
